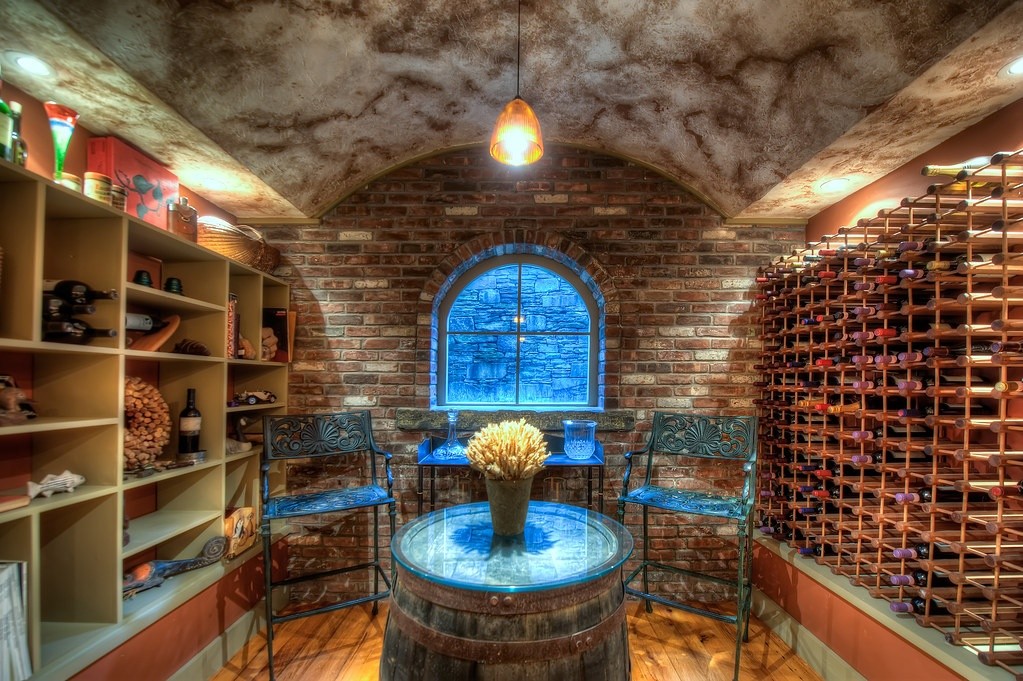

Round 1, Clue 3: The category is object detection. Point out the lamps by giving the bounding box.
[489,0,544,167]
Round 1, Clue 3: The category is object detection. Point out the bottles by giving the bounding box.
[434,408,467,460]
[9,100,28,167]
[179,388,203,453]
[0,80,14,163]
[43,293,95,320]
[750,155,1023,621]
[43,279,119,304]
[167,196,198,242]
[43,318,116,345]
[125,313,166,333]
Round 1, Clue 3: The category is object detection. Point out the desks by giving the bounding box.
[379,500,635,681]
[751,527,1023,681]
[417,435,605,514]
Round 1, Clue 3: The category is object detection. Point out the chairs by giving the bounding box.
[617,412,759,681]
[262,410,397,681]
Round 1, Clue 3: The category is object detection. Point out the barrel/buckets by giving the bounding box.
[378,502,632,681]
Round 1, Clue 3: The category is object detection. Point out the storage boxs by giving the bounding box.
[87,136,179,231]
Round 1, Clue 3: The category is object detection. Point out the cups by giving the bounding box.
[46,101,81,184]
[449,477,472,505]
[543,477,567,503]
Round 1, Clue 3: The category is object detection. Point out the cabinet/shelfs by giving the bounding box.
[0,158,290,681]
[759,149,1023,681]
